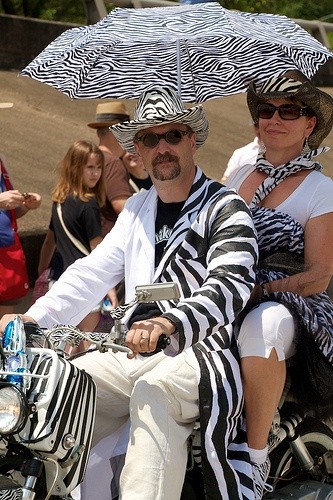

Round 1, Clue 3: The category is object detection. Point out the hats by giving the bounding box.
[108,86,209,157]
[247,69,333,150]
[88,102,130,129]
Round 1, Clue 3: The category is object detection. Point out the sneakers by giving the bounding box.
[251,455,274,500]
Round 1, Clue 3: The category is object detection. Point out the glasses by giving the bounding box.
[256,103,315,120]
[134,129,193,148]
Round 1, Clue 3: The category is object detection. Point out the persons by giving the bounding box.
[0,158,42,318]
[228,69,333,500]
[88,102,153,235]
[221,119,261,182]
[0,87,260,500]
[38,140,118,356]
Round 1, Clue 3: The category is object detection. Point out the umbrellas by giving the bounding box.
[18,2,333,97]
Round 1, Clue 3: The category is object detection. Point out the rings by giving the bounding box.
[141,336,149,340]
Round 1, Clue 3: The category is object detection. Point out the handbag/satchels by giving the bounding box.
[0,243,29,303]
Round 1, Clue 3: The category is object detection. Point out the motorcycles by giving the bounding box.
[0,282,333,500]
